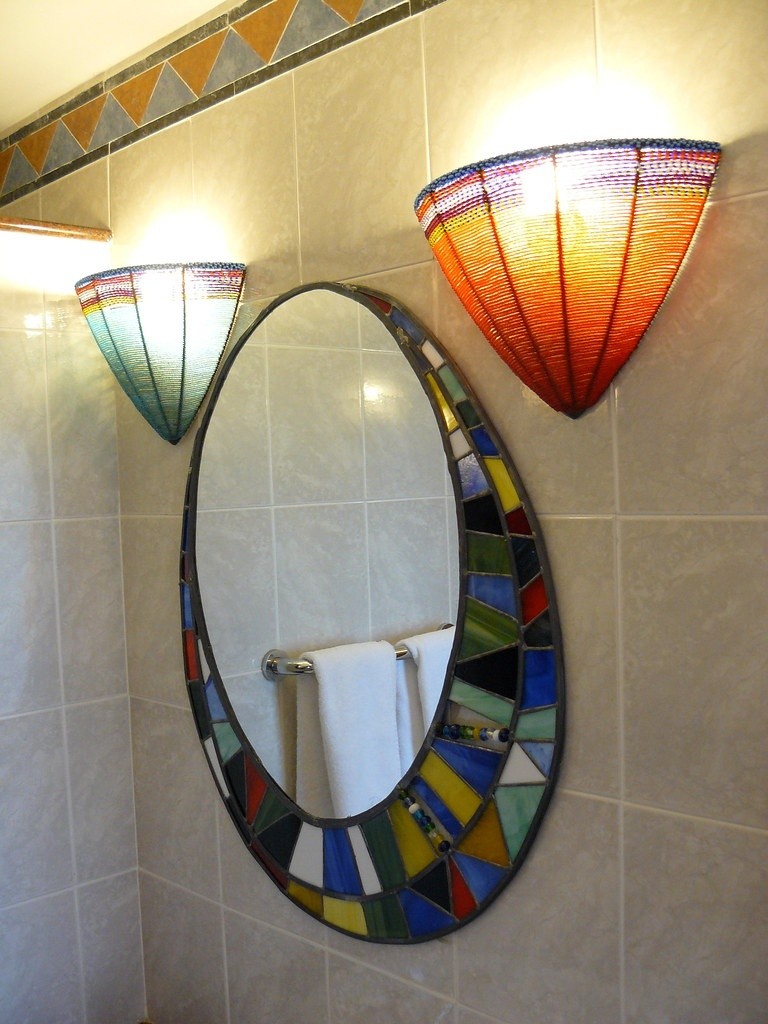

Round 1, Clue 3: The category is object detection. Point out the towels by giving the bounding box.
[295,640,403,819]
[396,626,467,778]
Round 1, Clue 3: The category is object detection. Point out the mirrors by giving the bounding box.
[181,284,565,944]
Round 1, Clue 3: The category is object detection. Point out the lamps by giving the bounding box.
[415,137,719,419]
[74,264,246,445]
